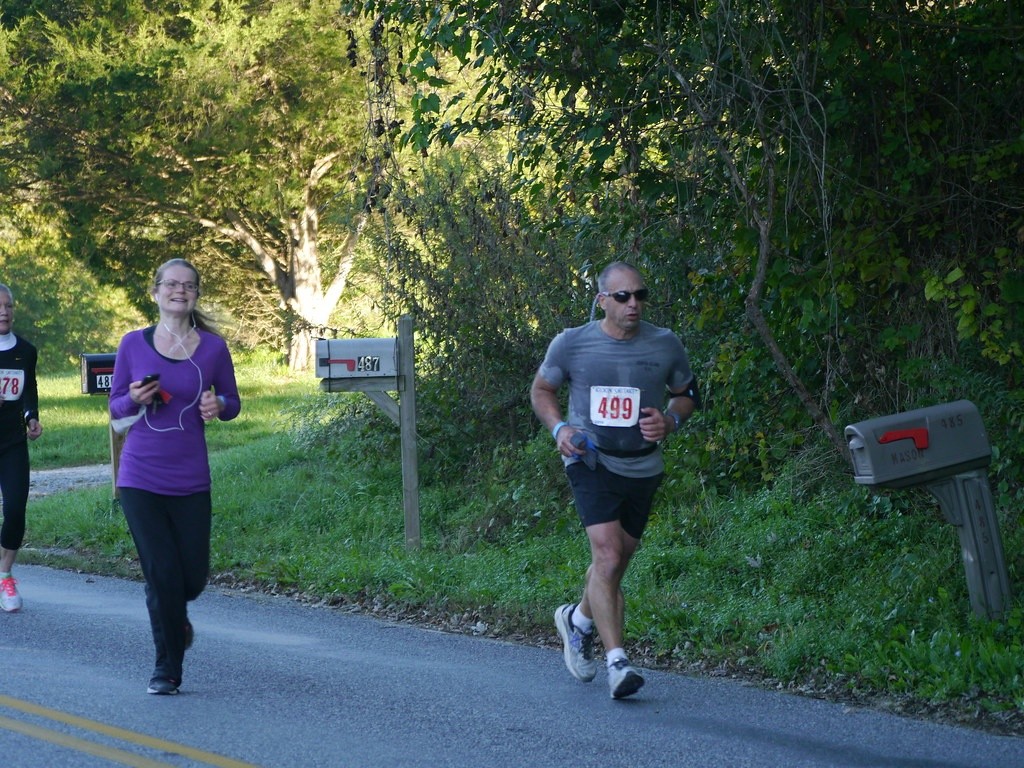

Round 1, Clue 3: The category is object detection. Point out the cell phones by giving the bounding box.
[140,373,161,388]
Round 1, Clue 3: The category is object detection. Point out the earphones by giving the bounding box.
[154,293,159,302]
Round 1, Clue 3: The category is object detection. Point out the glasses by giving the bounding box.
[601,289,648,303]
[157,279,199,292]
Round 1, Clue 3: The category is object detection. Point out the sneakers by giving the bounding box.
[183,617,193,649]
[147,676,179,695]
[0,575,23,613]
[606,657,645,699]
[554,603,597,682]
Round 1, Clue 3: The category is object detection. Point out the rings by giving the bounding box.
[208,412,213,417]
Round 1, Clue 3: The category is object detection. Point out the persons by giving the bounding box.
[0,285,43,612]
[530,261,698,700]
[110,258,241,695]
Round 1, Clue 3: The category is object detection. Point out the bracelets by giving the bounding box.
[26,413,39,426]
[553,422,569,439]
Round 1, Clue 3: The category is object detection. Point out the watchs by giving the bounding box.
[667,412,682,431]
